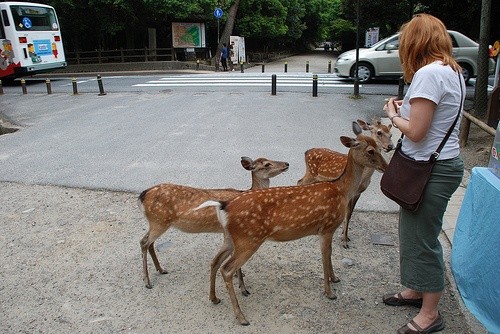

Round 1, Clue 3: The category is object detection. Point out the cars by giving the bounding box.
[333,29,496,84]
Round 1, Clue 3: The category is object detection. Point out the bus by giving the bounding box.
[0,1,68,83]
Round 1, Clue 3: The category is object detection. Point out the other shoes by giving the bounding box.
[383,292,423,308]
[397,310,445,334]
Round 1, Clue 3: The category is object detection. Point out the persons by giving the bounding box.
[220,43,235,72]
[324,43,335,55]
[382,14,466,334]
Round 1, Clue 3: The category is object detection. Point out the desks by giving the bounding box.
[450,166,500,334]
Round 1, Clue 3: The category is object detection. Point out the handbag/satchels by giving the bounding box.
[230,57,236,62]
[380,142,434,214]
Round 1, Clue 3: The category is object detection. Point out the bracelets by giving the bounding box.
[392,112,401,129]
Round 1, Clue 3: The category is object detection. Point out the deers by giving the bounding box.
[138,155,290,297]
[208,121,391,326]
[296,118,396,250]
